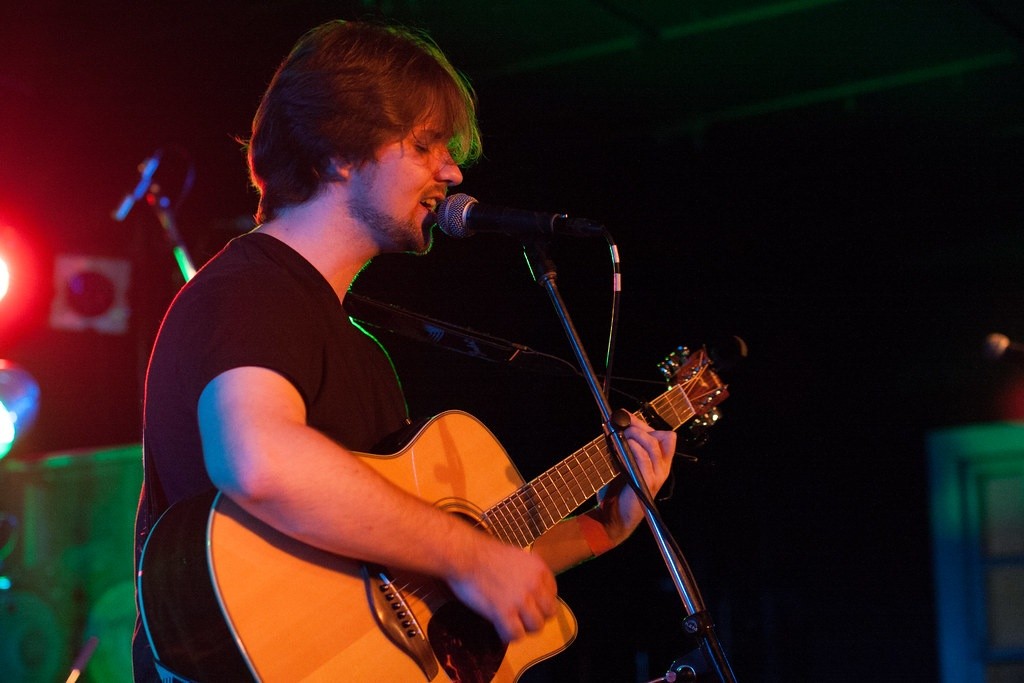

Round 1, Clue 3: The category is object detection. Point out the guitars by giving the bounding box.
[138,335,752,683]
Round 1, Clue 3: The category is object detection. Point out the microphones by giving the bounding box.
[437,193,605,239]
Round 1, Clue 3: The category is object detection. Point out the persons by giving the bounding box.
[130,20,678,683]
